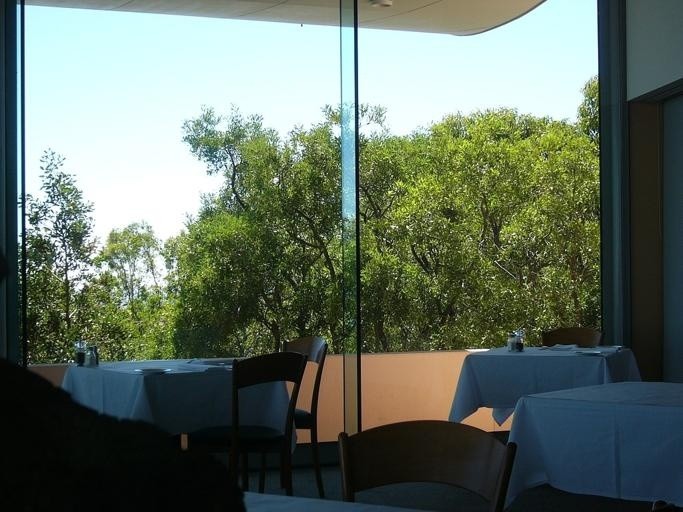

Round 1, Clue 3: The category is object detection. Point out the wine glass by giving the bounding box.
[73,341,86,367]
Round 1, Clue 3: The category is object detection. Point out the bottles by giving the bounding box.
[85,346,100,369]
[508,331,524,353]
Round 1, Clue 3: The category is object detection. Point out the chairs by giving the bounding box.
[339,420,517,512]
[283,336,328,499]
[183,352,307,498]
[542,327,604,346]
